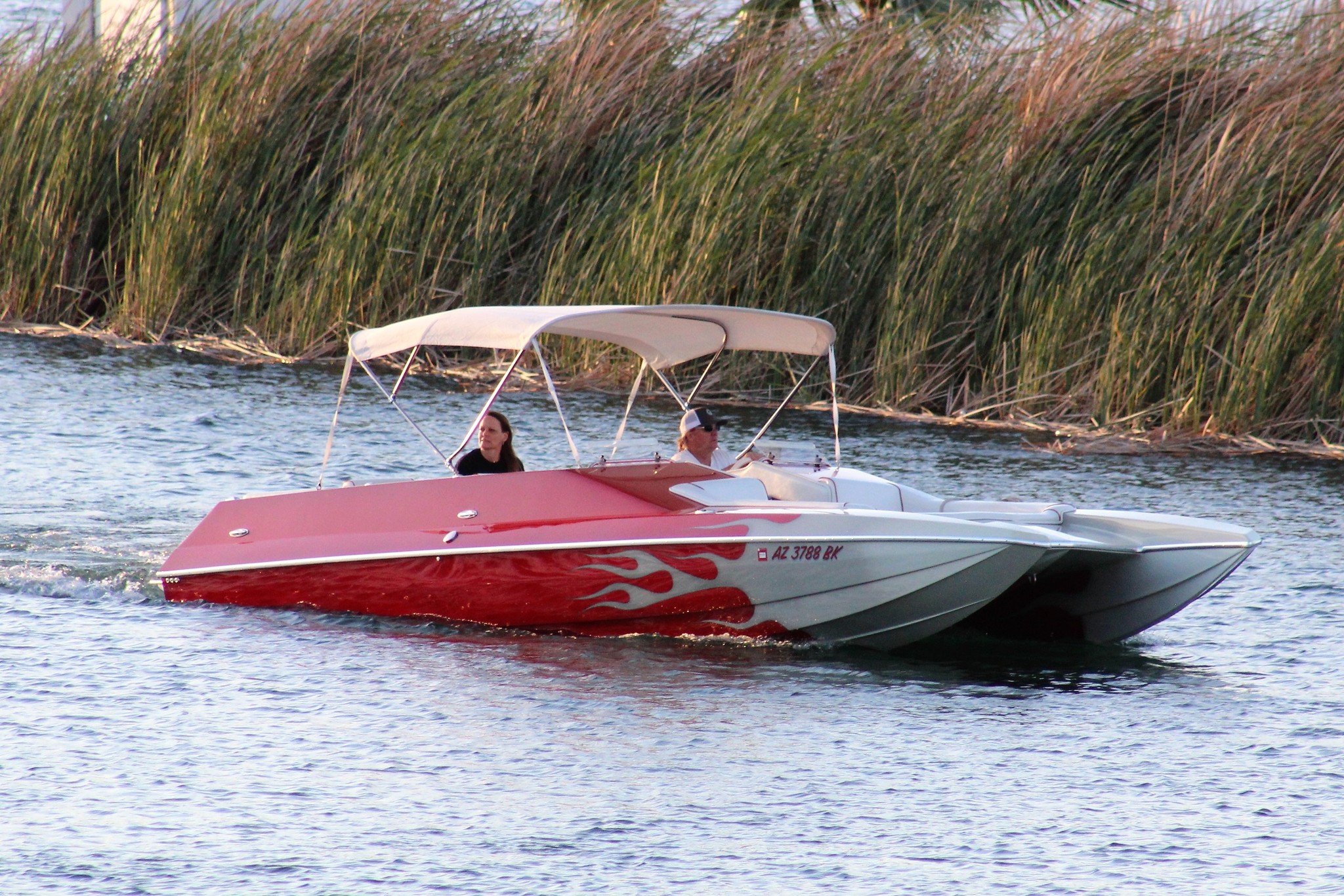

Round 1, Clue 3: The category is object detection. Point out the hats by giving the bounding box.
[679,407,728,438]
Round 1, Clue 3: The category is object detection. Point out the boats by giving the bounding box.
[154,304,1265,660]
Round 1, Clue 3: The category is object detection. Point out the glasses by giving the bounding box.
[697,424,720,432]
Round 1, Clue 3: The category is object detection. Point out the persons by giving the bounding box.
[670,408,761,470]
[455,412,525,476]
